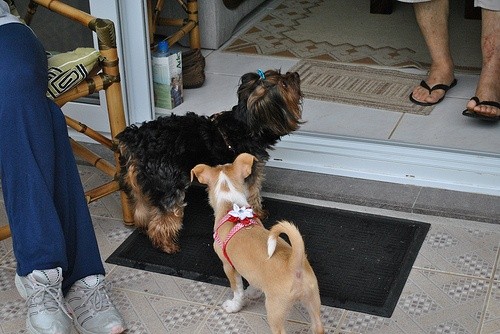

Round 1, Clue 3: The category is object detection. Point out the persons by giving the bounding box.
[397,0,500,119]
[0,0,128,334]
[170,77,184,107]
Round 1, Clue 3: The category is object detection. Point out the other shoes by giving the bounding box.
[182,48,206,89]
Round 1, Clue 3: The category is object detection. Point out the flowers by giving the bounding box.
[225,203,259,225]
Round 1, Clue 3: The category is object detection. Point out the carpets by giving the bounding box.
[220,0,482,74]
[104,184,431,320]
[288,58,436,116]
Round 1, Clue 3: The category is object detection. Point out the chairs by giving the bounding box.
[0,0,134,243]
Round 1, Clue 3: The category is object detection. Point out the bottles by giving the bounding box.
[151,40,184,110]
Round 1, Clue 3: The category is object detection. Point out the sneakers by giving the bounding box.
[65,274,126,334]
[15,267,73,334]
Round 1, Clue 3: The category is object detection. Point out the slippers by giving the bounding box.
[410,78,458,106]
[462,97,500,121]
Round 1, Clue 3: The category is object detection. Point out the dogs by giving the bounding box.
[190,153,324,334]
[110,70,309,256]
